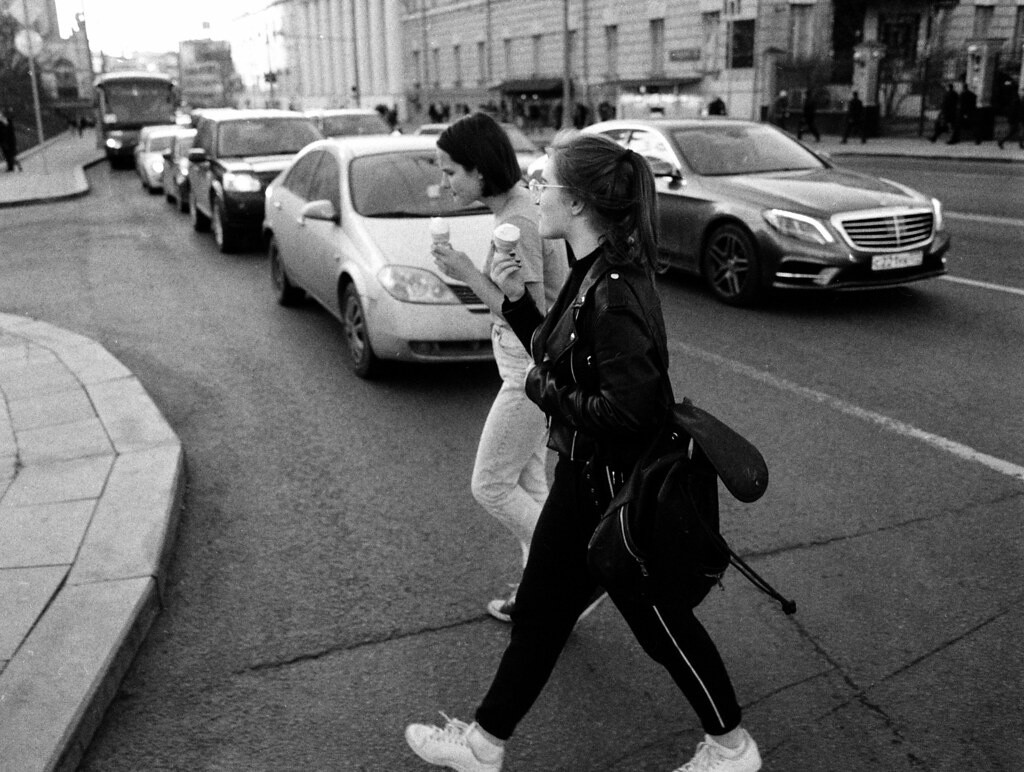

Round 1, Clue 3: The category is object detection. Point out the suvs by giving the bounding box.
[189,107,393,254]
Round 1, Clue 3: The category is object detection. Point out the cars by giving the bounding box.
[527,118,954,307]
[260,119,574,381]
[133,123,197,212]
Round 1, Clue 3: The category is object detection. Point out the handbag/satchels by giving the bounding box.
[586,395,769,611]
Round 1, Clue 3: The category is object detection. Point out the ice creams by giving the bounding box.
[428,217,450,248]
[492,224,522,254]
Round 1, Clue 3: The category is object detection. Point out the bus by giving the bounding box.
[90,72,182,170]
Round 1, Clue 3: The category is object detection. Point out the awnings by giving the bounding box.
[489,77,571,93]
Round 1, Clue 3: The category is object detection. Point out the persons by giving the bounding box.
[707,99,728,116]
[428,100,470,124]
[0,111,23,172]
[926,83,1024,150]
[404,132,762,772]
[68,113,85,138]
[840,90,866,144]
[479,97,616,131]
[769,89,790,129]
[431,113,609,633]
[375,103,404,134]
[796,90,821,142]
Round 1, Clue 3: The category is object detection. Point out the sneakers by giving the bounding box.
[671,729,763,772]
[487,582,520,621]
[405,711,505,772]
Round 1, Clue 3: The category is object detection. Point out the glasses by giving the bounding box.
[529,176,577,204]
